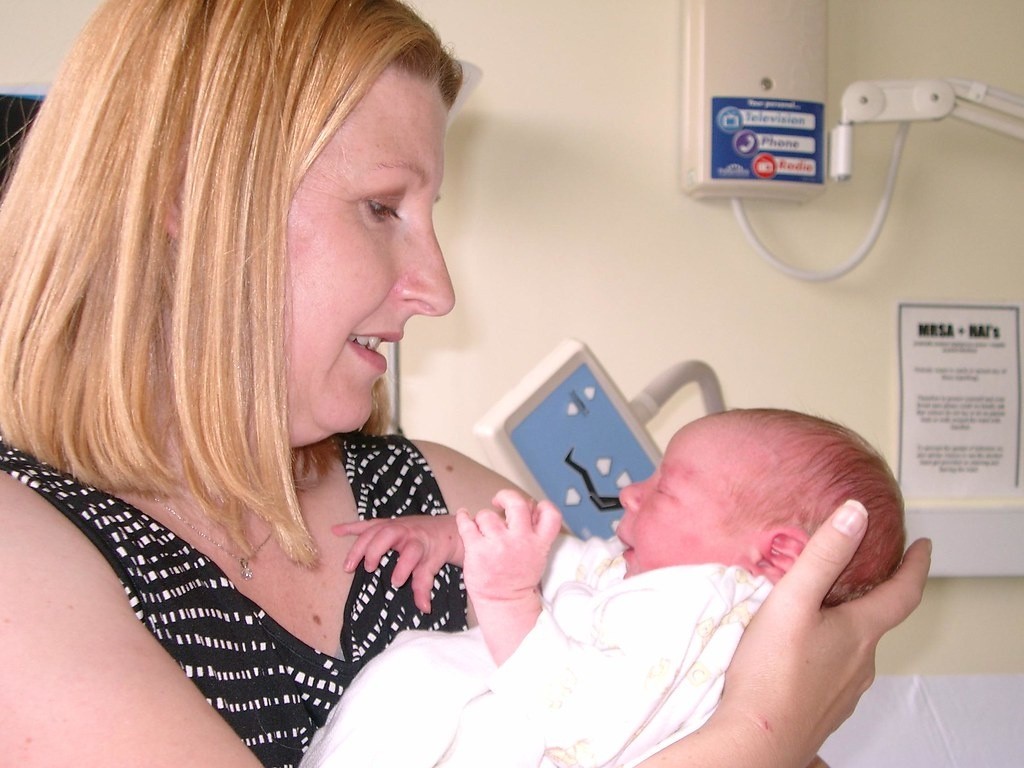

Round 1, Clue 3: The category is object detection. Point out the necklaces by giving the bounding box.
[152,497,275,581]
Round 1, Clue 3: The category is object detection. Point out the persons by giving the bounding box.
[298,407,906,767]
[0,1,933,768]
